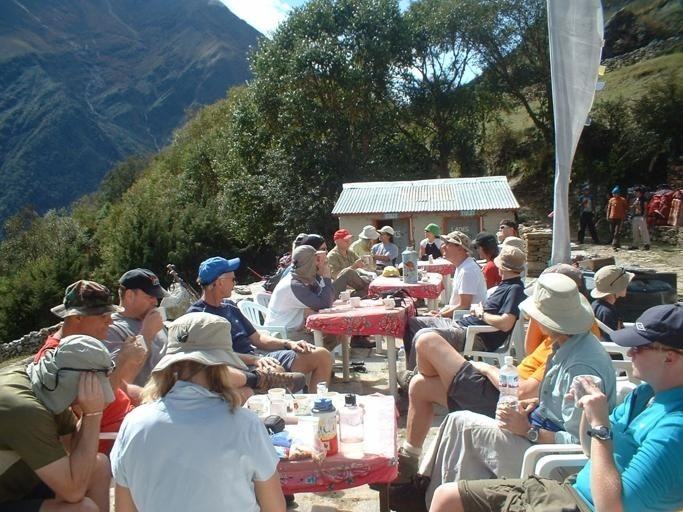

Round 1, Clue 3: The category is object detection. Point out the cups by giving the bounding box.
[381,297,405,309]
[246,388,309,411]
[496,232,504,244]
[338,291,361,307]
[573,374,603,408]
[362,254,370,266]
[153,307,167,324]
[132,333,151,353]
[421,266,429,282]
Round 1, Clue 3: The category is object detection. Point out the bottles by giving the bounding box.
[309,380,365,458]
[402,245,419,284]
[497,356,519,410]
[428,254,434,264]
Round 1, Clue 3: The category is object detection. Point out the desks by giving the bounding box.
[417,258,455,305]
[368,272,444,355]
[306,293,418,401]
[239,394,398,511]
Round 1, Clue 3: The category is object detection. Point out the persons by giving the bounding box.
[576,185,600,244]
[628,188,650,250]
[604,185,630,246]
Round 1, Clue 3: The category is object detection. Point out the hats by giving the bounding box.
[31,332,120,413]
[517,272,596,336]
[51,279,125,321]
[424,220,526,273]
[152,308,251,374]
[291,225,396,270]
[590,266,636,299]
[118,269,168,298]
[612,187,622,194]
[197,255,240,286]
[609,304,683,352]
[523,263,585,297]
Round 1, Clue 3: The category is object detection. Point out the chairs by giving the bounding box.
[451,305,646,483]
[237,292,292,341]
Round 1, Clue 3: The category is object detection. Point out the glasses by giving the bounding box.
[631,342,669,353]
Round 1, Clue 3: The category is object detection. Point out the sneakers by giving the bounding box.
[396,367,414,394]
[250,369,307,396]
[369,445,428,512]
